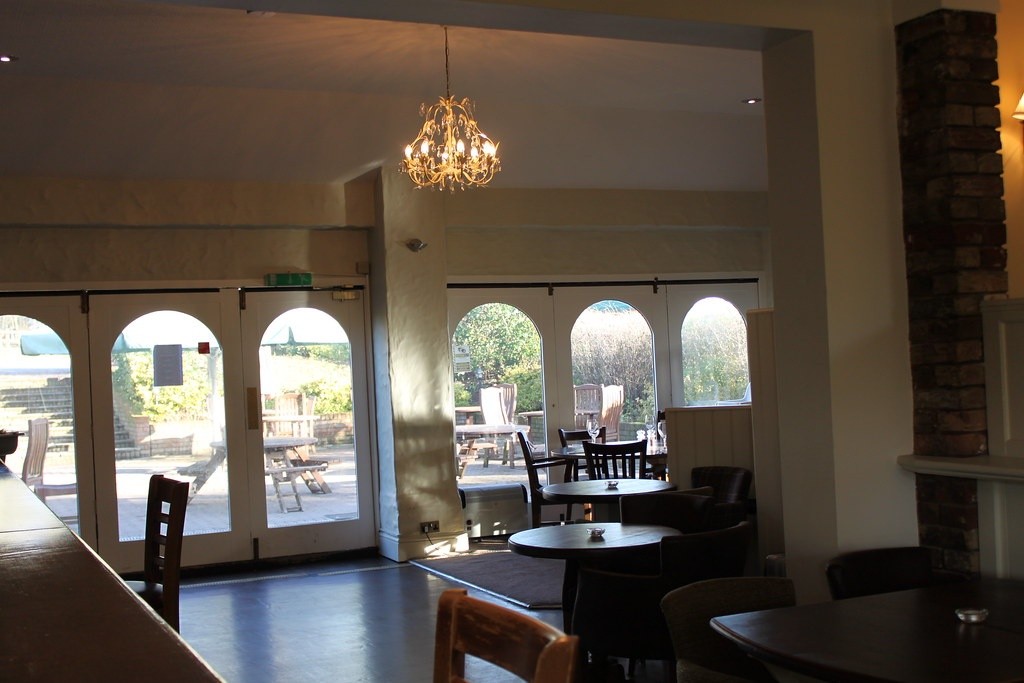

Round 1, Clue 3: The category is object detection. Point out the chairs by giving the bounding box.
[434,383,780,683]
[21,416,50,500]
[826,545,934,598]
[263,392,317,436]
[120,473,189,633]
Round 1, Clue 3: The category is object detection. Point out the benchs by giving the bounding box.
[176,456,332,506]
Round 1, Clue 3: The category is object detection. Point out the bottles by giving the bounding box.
[637,430,645,442]
[646,430,657,455]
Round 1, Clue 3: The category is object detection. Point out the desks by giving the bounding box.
[551,441,667,478]
[1,456,72,533]
[0,528,226,683]
[508,524,684,629]
[709,579,1024,682]
[541,478,677,523]
[453,424,531,469]
[208,438,321,513]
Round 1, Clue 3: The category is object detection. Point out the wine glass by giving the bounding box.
[645,413,667,453]
[586,419,600,443]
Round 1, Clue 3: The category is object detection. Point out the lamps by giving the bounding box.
[398,24,501,193]
